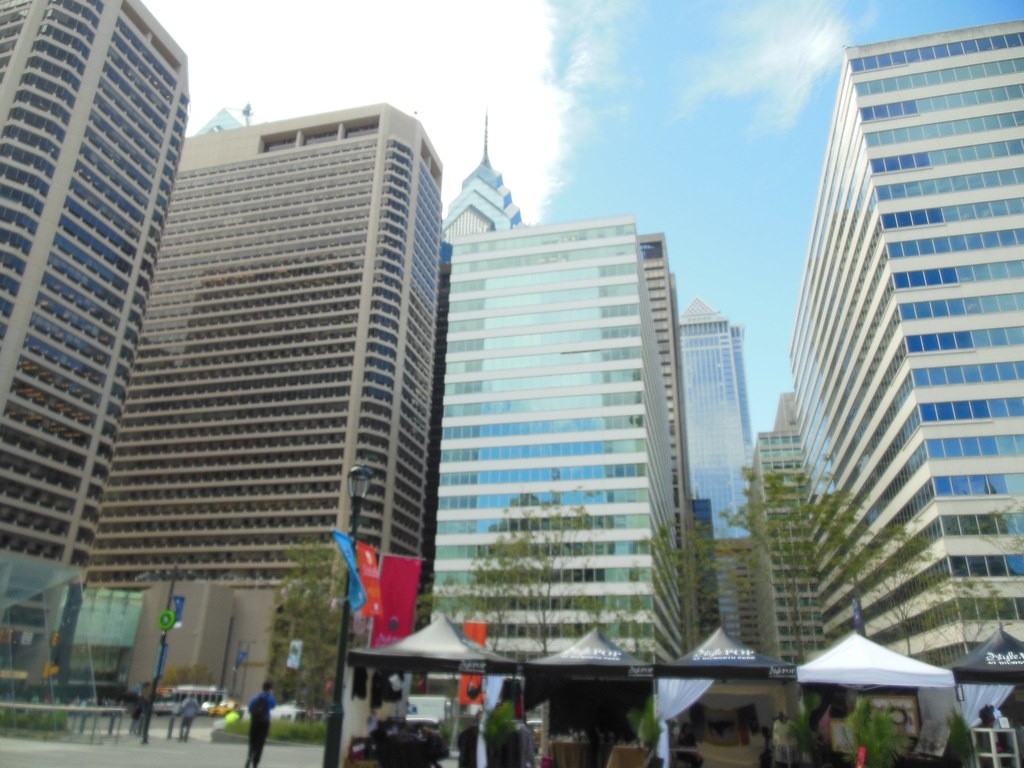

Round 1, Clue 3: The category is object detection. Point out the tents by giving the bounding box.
[356,614,1022,768]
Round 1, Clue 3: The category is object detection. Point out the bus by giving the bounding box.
[154,685,230,716]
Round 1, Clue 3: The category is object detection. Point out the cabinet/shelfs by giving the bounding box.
[971,727,1021,768]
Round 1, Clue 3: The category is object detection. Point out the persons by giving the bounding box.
[245,679,276,768]
[129,704,143,736]
[176,690,199,742]
[772,710,800,768]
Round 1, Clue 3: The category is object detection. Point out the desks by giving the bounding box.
[548,740,591,768]
[606,746,650,768]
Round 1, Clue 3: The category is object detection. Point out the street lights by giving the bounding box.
[321,460,376,768]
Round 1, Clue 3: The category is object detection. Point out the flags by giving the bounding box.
[331,529,422,652]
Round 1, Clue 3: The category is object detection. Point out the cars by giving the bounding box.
[271,704,335,723]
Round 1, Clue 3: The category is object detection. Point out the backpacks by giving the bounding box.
[253,700,268,726]
